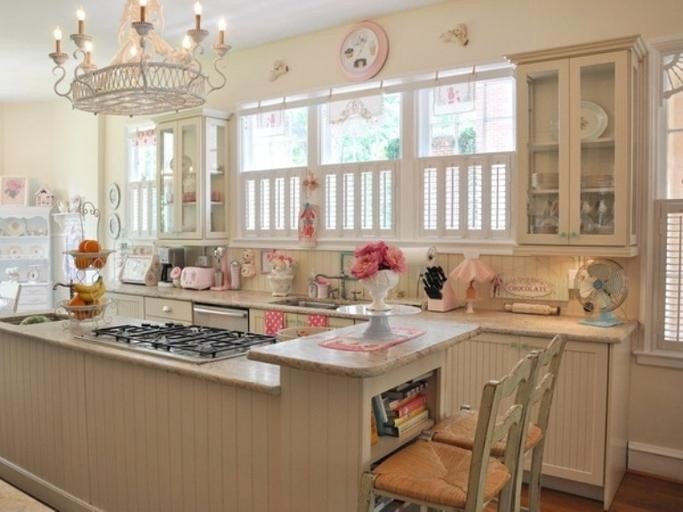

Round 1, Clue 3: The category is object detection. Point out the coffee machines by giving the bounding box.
[157,247,185,288]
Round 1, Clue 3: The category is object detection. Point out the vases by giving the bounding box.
[363,270,399,312]
[270,276,293,296]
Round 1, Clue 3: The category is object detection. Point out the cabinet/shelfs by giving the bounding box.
[454,331,635,511]
[111,293,144,320]
[142,296,193,327]
[0,203,56,315]
[156,117,233,242]
[512,48,642,248]
[249,308,354,335]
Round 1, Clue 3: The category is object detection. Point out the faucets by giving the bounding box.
[52,279,74,299]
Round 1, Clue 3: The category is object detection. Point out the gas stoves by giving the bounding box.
[72,321,278,365]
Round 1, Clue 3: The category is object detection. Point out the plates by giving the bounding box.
[580,100,609,141]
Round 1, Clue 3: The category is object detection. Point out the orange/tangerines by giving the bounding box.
[70,294,87,306]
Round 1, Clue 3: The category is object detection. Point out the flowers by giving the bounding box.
[266,251,297,275]
[350,239,408,279]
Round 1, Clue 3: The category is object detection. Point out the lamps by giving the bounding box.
[46,0,230,118]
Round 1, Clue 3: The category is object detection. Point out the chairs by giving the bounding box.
[363,330,569,510]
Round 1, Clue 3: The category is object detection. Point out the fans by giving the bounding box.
[574,256,630,328]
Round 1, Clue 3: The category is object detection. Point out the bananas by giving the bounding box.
[72,277,105,301]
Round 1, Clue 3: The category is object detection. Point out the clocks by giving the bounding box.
[338,20,391,82]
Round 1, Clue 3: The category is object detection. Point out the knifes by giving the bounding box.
[422,266,447,300]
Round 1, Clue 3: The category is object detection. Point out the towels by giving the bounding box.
[308,312,328,328]
[263,309,285,336]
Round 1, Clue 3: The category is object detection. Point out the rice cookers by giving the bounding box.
[180,263,217,290]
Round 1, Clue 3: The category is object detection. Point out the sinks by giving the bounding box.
[0,313,68,326]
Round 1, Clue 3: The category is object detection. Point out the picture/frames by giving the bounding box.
[0,175,27,205]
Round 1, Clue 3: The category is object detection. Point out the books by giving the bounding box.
[368,369,439,457]
